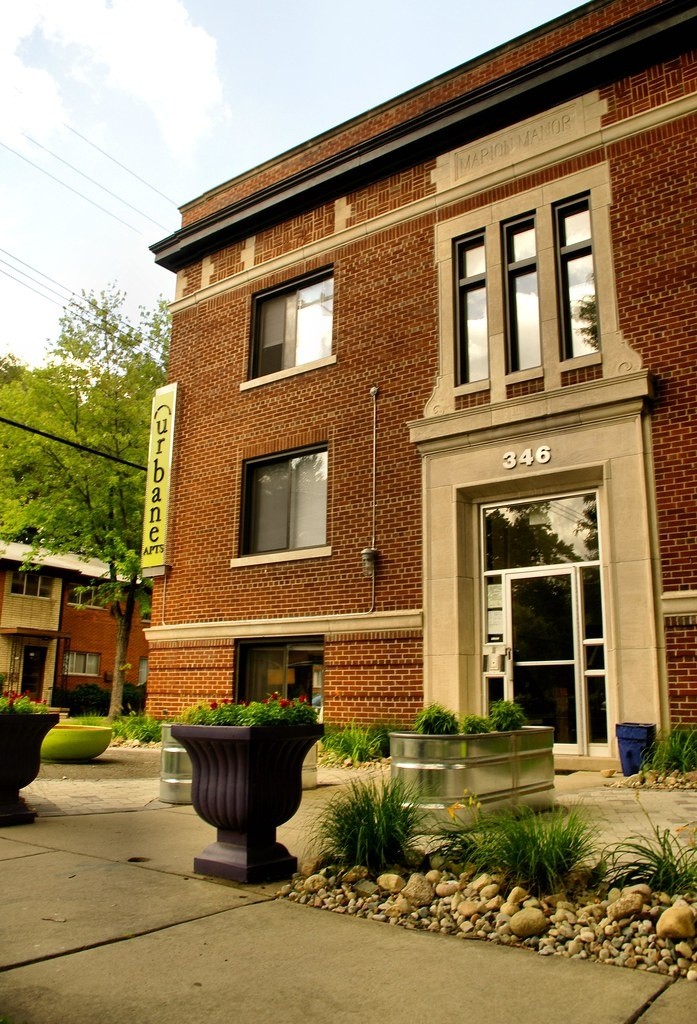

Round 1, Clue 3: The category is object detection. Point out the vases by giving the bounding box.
[1,714,60,827]
[171,724,325,884]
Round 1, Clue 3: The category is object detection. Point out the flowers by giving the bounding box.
[0,690,49,714]
[172,693,320,725]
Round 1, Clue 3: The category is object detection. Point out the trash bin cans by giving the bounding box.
[615,721,657,776]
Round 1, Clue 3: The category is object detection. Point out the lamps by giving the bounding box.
[360,549,377,579]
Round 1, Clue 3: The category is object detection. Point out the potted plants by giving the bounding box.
[388,697,556,834]
